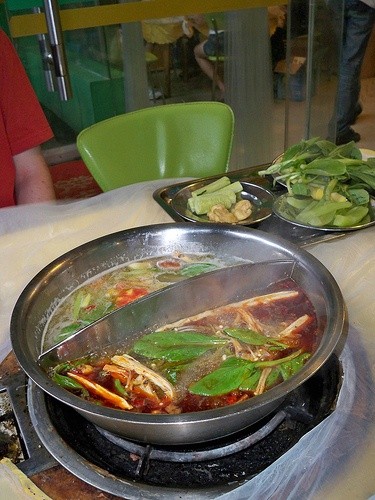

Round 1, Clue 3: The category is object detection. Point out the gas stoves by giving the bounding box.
[0,336,355,499]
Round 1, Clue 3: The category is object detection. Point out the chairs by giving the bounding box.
[105,30,177,105]
[207,12,279,100]
[76,101,235,194]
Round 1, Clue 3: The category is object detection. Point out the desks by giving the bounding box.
[0,149,375,500]
[142,4,287,98]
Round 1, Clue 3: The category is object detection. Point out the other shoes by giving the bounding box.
[327,129,360,145]
[349,104,362,124]
[148,87,162,100]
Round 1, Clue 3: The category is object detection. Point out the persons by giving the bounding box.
[325,0,375,142]
[193,31,228,101]
[0,29,54,210]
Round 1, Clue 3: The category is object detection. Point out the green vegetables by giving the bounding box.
[258,136,374,227]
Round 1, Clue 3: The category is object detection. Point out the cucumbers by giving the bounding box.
[188,176,244,215]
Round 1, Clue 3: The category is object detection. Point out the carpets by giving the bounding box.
[47,158,104,201]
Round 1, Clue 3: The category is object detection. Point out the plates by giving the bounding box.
[271,191,375,230]
[171,179,276,225]
[272,152,292,187]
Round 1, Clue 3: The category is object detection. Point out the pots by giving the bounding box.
[9,222,345,444]
